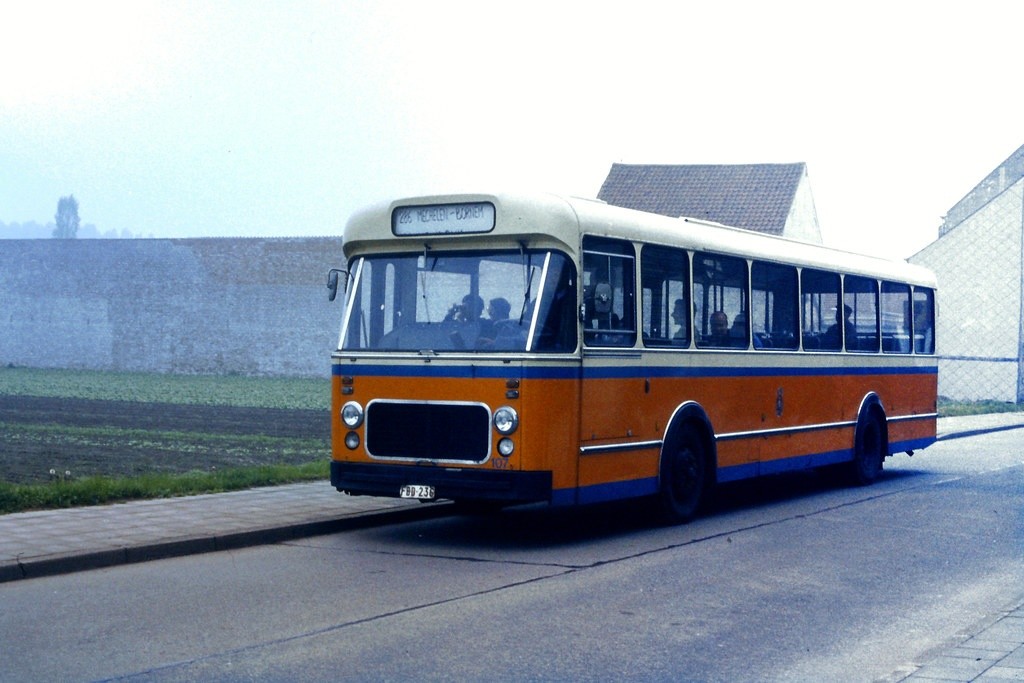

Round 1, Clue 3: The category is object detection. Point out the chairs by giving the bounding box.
[758,331,925,352]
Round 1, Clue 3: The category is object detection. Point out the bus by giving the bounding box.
[326,186,940,524]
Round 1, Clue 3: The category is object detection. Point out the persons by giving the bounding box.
[730,311,763,348]
[587,267,616,337]
[822,304,858,349]
[709,310,730,338]
[443,294,512,340]
[671,298,700,340]
[523,256,573,333]
